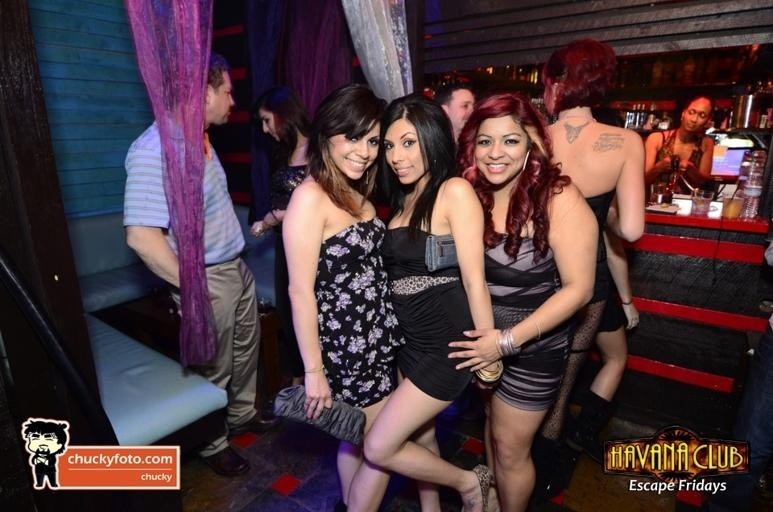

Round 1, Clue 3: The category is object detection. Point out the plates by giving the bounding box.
[645,206,679,214]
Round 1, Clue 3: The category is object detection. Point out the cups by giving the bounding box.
[689,188,715,215]
[720,191,744,219]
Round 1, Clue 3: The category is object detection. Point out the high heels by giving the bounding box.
[473,465,498,512]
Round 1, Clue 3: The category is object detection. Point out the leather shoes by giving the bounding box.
[202,446,250,477]
[231,411,281,436]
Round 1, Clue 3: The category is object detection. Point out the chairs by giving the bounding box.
[69,208,281,456]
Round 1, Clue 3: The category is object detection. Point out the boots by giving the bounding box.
[566,387,610,463]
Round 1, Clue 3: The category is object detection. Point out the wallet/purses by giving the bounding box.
[425,233,459,272]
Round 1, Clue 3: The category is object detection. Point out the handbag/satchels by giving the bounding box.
[271,385,366,445]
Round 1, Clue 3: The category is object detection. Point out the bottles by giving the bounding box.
[629,102,657,128]
[739,176,763,219]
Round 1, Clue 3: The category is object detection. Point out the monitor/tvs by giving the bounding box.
[699,133,756,181]
